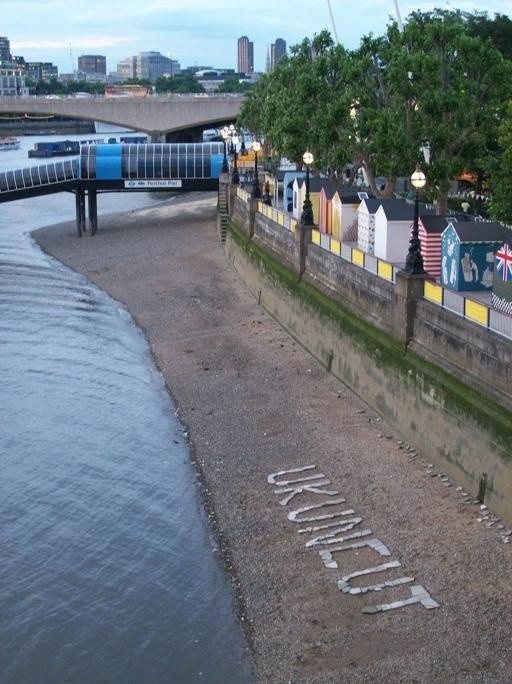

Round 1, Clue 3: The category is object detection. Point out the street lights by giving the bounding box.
[167,51,176,96]
[299,146,316,225]
[401,162,427,274]
[220,124,262,198]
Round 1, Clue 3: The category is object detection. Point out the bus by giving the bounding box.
[105,84,159,97]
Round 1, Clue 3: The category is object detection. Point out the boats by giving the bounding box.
[28,137,149,158]
[0,137,21,150]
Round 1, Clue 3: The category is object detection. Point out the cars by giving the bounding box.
[46,92,96,100]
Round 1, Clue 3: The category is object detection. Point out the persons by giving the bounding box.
[244,168,248,179]
[249,166,254,180]
[263,190,272,206]
[265,180,270,196]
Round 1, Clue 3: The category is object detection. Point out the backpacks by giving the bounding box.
[467,207,472,215]
